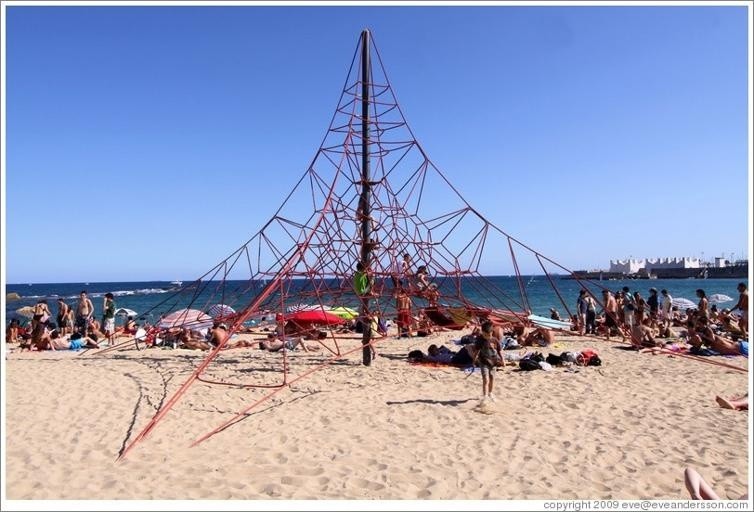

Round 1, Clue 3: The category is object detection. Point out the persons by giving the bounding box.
[717,392,750,411]
[683,467,748,500]
[8,195,749,370]
[471,322,506,403]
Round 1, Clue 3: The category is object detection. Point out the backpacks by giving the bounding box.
[519,350,602,372]
